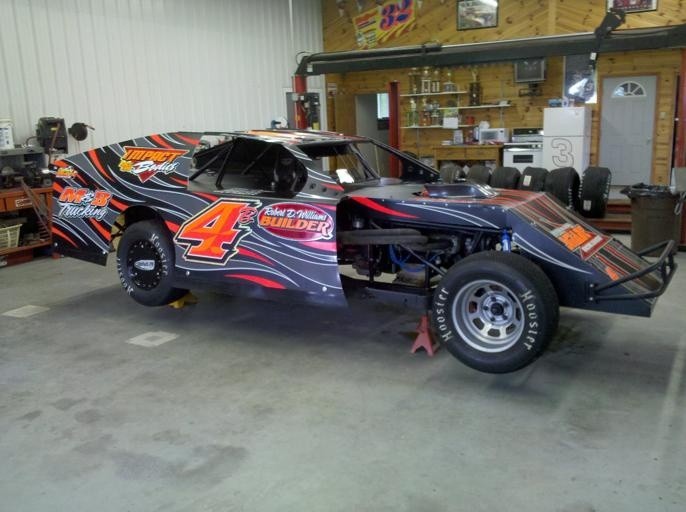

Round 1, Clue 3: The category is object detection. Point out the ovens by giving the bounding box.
[502,149,543,174]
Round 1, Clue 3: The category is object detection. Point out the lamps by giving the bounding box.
[586,49,598,72]
[594,7,625,41]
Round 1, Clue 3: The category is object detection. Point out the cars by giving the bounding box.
[52,131,677,373]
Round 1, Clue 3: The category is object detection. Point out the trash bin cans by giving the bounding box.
[620,184,685,257]
[271,120,281,129]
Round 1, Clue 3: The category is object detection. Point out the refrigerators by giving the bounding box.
[542,106,593,199]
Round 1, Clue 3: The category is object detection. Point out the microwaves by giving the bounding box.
[478,128,510,143]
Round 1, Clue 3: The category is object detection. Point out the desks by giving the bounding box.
[431,142,503,170]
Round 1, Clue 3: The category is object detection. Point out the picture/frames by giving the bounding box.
[457,0,498,30]
[606,0,657,14]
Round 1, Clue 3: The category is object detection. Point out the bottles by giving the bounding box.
[404,66,457,127]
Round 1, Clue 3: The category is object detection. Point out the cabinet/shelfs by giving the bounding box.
[0,147,46,188]
[0,186,53,265]
[399,90,514,129]
